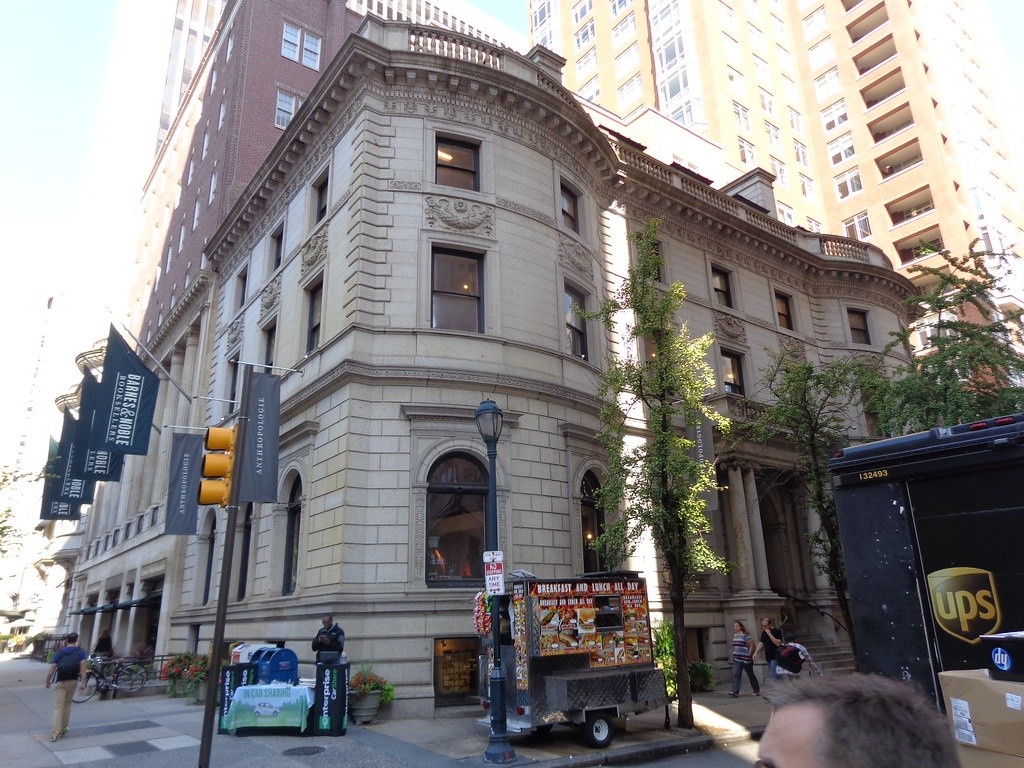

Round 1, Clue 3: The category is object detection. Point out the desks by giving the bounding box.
[230,682,313,734]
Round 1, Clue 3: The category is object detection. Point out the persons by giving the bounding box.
[752,616,784,680]
[310,614,346,670]
[6,630,26,654]
[728,619,762,697]
[93,628,113,660]
[45,631,88,742]
[773,631,817,685]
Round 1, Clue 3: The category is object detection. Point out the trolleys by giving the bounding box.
[463,566,666,749]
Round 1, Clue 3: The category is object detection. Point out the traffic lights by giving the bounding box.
[195,423,239,507]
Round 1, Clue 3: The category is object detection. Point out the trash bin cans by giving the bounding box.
[249,647,268,663]
[256,647,298,685]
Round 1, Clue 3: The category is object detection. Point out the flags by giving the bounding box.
[90,321,163,460]
[69,361,127,485]
[38,433,83,521]
[52,403,98,504]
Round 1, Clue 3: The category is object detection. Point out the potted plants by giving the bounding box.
[347,688,382,726]
[173,675,196,697]
[198,678,224,706]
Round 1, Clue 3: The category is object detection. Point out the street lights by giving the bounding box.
[475,398,517,766]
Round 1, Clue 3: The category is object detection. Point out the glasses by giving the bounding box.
[321,621,331,625]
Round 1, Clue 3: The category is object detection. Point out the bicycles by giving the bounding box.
[74,652,148,705]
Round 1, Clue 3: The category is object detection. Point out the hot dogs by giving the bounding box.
[558,633,577,647]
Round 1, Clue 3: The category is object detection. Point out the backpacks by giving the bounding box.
[57,648,81,681]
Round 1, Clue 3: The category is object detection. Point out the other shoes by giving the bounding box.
[752,692,760,696]
[51,731,65,742]
[728,690,739,698]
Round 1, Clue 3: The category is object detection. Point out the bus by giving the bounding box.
[827,412,1023,764]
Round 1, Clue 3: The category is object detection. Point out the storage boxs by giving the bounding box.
[937,667,1024,768]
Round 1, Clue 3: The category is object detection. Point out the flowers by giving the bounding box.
[158,653,211,682]
[348,671,394,703]
